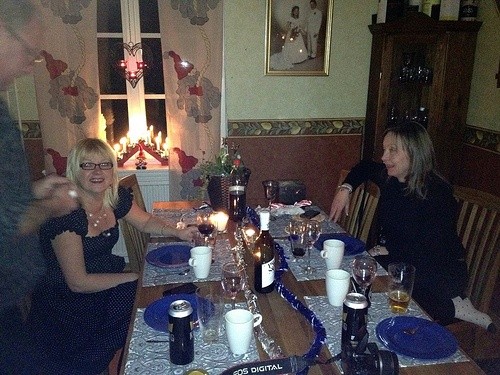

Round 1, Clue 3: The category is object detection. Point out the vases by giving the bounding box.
[207,175,232,211]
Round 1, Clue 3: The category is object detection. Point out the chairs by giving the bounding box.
[118,174,148,275]
[445,185,494,365]
[335,169,381,244]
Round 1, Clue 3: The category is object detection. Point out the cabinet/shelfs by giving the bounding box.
[363,20,483,188]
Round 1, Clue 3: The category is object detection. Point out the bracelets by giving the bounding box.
[337,185,353,194]
[161,224,168,235]
[373,245,380,256]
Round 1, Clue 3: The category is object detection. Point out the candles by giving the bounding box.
[113,124,168,159]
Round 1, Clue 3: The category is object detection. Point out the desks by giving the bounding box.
[119,198,486,375]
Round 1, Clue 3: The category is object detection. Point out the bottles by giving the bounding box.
[228,159,247,221]
[253,210,275,294]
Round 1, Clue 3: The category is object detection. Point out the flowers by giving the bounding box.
[196,144,241,198]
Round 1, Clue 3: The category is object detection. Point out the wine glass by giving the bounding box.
[196,210,217,264]
[288,220,321,275]
[352,255,378,296]
[222,262,247,311]
[263,180,278,213]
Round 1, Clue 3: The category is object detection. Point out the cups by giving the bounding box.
[188,246,212,279]
[225,309,263,354]
[320,239,346,270]
[196,284,227,345]
[325,269,350,307]
[387,263,416,313]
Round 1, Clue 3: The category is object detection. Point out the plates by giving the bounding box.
[146,245,194,269]
[376,316,457,360]
[144,293,215,331]
[313,233,366,256]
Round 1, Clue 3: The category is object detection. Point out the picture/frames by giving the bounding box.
[264,0,334,76]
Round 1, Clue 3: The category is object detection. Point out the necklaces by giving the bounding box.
[87,211,107,227]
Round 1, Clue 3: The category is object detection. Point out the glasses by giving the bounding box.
[5,22,45,64]
[79,162,113,170]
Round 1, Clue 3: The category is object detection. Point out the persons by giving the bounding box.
[327,122,496,335]
[282,6,308,64]
[0,0,76,375]
[304,0,323,58]
[28,139,199,375]
